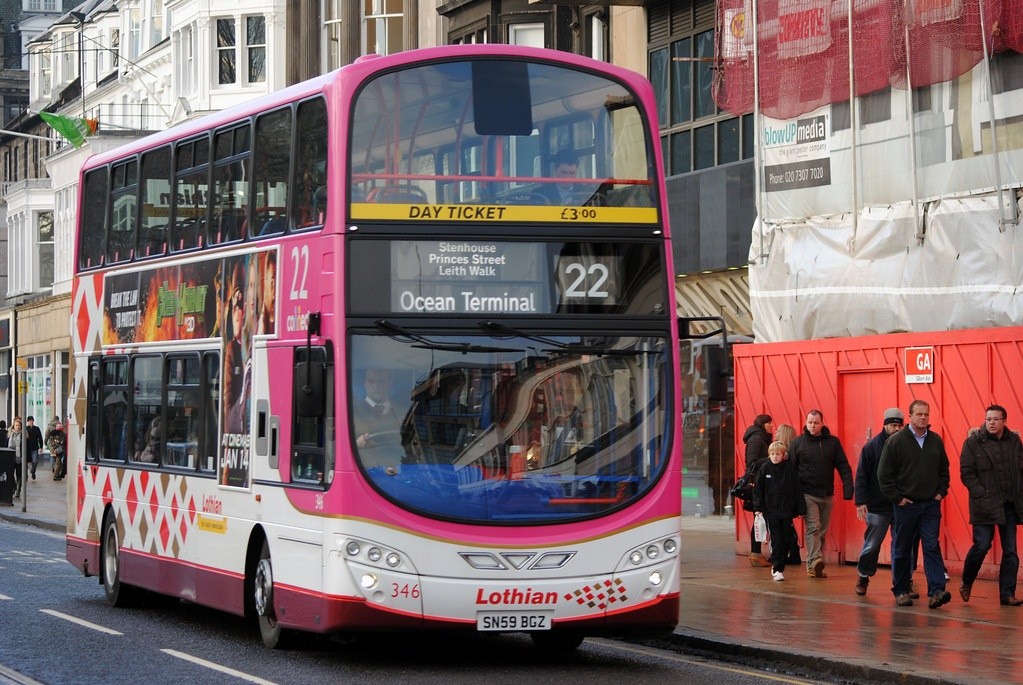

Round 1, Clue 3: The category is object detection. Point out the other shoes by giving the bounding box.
[750,552,763,567]
[785,556,802,565]
[855,574,870,595]
[813,558,825,579]
[1000,596,1023,607]
[54,476,62,481]
[944,572,951,581]
[59,474,64,479]
[762,552,772,567]
[30,471,36,480]
[771,565,785,581]
[807,570,827,579]
[896,594,913,607]
[960,580,974,602]
[909,579,919,599]
[927,591,951,609]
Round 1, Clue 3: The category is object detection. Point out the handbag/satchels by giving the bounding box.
[753,511,767,543]
[729,461,756,500]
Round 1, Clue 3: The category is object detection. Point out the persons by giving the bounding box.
[959,405,1023,605]
[354,368,526,480]
[708,409,854,581]
[104,392,217,470]
[529,147,586,205]
[854,408,950,598]
[0,415,66,498]
[209,251,276,487]
[876,400,951,609]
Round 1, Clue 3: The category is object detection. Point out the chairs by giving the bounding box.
[85,182,425,270]
[502,192,612,208]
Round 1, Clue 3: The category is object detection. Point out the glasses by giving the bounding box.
[985,416,1004,423]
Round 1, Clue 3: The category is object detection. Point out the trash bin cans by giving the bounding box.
[0,448,17,506]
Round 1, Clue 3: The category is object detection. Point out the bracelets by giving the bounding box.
[136,449,140,451]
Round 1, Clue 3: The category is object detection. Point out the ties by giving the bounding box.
[374,404,384,417]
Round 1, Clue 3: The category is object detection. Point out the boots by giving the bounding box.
[15,483,21,497]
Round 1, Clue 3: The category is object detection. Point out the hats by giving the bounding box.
[55,422,64,431]
[27,416,34,422]
[0,421,6,428]
[884,408,904,426]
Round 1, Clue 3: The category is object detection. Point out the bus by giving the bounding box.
[62,42,734,652]
